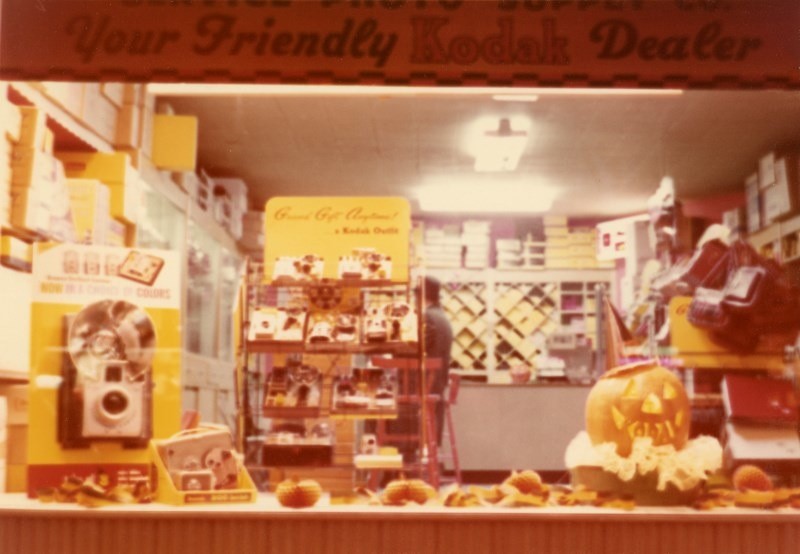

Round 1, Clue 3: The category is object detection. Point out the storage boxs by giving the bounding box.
[740,158,791,230]
[425,223,614,268]
[2,83,269,270]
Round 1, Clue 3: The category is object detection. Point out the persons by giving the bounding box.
[412,276,452,445]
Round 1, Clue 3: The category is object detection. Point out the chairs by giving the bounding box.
[372,359,461,487]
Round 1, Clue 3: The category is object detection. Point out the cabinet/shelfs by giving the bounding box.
[241,259,425,494]
[424,273,616,379]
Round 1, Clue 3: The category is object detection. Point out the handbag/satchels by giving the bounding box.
[688,244,791,352]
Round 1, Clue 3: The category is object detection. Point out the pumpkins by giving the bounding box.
[385,472,429,506]
[732,465,774,491]
[500,465,542,496]
[276,473,323,509]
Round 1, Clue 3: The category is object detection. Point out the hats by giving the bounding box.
[416,275,443,300]
